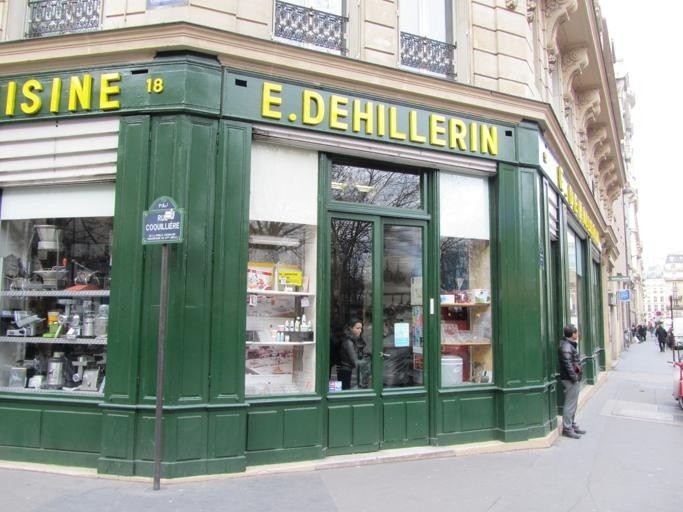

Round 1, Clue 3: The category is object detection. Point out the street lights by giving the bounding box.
[621,188,636,344]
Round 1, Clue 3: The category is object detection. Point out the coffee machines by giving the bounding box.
[33,223,72,291]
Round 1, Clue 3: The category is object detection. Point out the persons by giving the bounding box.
[336,317,369,390]
[631,320,673,353]
[558,326,586,439]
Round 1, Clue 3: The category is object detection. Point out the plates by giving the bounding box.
[2,254,21,277]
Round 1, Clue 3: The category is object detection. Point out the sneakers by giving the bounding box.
[562,426,586,439]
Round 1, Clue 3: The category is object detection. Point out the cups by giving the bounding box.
[474,289,488,303]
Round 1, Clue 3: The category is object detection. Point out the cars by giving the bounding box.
[662,319,683,349]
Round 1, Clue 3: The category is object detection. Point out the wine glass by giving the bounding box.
[454,277,464,290]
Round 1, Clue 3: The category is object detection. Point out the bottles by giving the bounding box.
[43,298,108,390]
[274,315,312,344]
